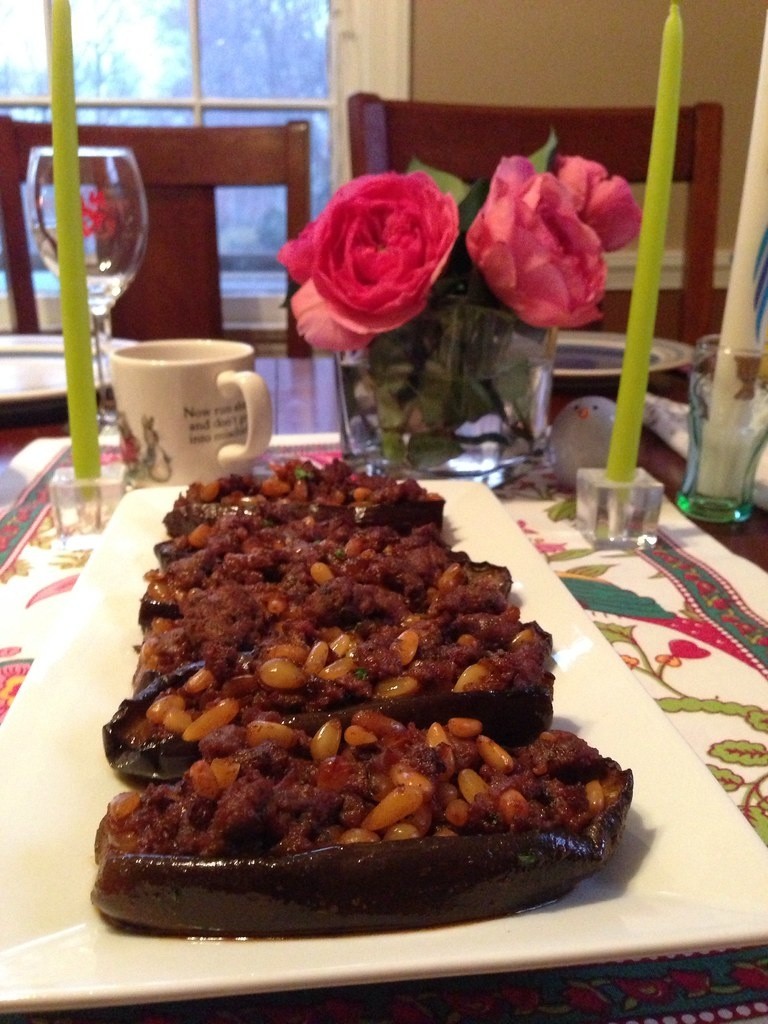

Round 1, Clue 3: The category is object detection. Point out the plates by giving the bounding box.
[0,335,140,415]
[553,329,694,394]
[0,478,768,1018]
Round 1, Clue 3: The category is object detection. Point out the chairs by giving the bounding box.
[0,115,312,358]
[346,89,728,370]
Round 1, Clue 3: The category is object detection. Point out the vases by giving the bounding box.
[343,284,557,490]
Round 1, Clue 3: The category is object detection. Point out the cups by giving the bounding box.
[111,340,274,494]
[676,335,768,524]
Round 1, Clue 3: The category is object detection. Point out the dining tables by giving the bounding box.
[0,355,768,1023]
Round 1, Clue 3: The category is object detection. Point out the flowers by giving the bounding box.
[276,146,644,351]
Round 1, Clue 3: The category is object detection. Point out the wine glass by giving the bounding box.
[27,147,150,438]
